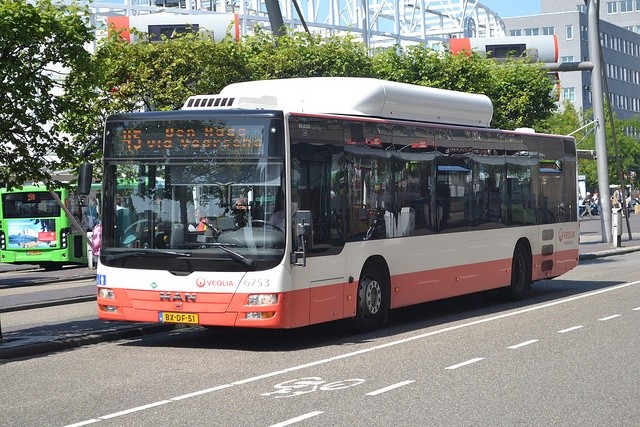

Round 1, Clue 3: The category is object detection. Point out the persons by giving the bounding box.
[581,191,597,219]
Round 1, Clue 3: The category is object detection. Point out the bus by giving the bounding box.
[95,77,579,329]
[0,178,167,271]
